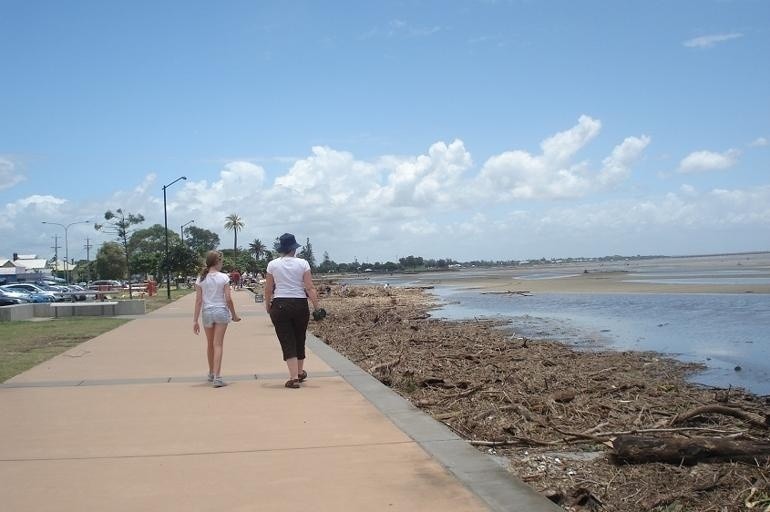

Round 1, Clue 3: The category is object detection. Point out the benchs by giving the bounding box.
[50,302,118,317]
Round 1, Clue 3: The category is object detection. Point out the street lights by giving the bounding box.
[40,220,90,283]
[160,176,188,299]
[180,219,195,244]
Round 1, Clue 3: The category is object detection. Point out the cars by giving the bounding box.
[0,278,127,307]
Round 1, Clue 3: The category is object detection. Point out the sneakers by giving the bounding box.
[298,371,307,380]
[286,379,299,388]
[208,374,227,387]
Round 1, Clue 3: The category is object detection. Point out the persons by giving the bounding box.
[193,250,241,387]
[265,233,323,388]
[227,269,253,292]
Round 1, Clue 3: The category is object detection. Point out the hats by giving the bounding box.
[277,233,301,252]
